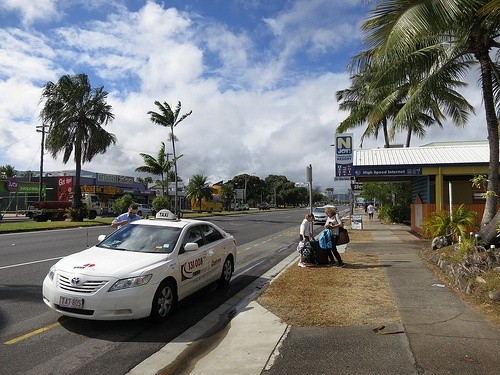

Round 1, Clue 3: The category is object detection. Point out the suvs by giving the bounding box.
[136,203,156,216]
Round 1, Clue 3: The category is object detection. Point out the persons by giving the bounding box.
[111,204,140,238]
[367,202,374,222]
[324,207,345,268]
[298,213,315,267]
[364,203,367,212]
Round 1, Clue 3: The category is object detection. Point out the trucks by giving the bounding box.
[18,193,103,221]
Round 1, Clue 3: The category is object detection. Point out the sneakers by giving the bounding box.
[326,261,336,267]
[298,263,306,268]
[336,263,345,268]
[302,261,306,264]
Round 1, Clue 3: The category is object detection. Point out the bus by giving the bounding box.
[191,194,224,213]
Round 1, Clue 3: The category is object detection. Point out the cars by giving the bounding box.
[312,204,338,224]
[42,209,238,320]
[225,202,270,211]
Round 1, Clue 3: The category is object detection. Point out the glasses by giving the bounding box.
[128,209,136,214]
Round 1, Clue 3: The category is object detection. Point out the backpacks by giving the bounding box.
[302,243,316,264]
[319,228,332,249]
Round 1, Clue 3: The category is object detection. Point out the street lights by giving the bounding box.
[35,123,50,202]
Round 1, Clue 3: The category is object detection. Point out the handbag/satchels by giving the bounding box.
[335,227,350,245]
